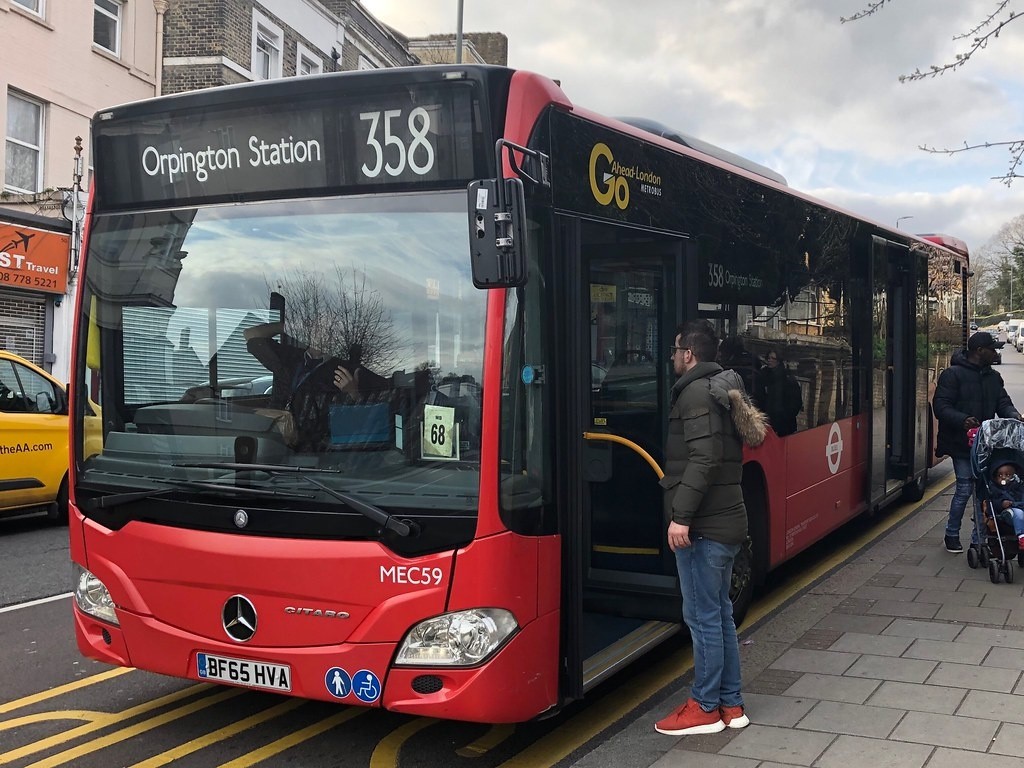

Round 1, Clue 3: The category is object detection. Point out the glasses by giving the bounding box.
[766,357,777,361]
[670,346,688,353]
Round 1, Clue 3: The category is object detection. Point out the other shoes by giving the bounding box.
[1018,537,1024,549]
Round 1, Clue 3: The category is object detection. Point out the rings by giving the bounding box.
[337,378,341,383]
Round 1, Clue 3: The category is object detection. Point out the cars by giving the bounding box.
[0,348,103,526]
[984,317,1024,354]
[970,321,979,330]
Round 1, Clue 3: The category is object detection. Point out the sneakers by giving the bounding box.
[654,697,749,736]
[943,534,963,552]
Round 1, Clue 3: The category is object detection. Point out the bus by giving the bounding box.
[64,62,975,725]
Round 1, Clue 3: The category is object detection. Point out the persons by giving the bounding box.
[241,287,386,452]
[934,331,1024,553]
[759,344,803,436]
[654,320,752,738]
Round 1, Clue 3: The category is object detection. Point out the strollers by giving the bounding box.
[967,418,1024,583]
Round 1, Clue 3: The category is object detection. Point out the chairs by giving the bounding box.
[592,349,660,425]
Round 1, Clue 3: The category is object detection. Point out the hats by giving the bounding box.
[968,331,1005,351]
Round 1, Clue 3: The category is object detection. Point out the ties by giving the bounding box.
[305,353,324,373]
